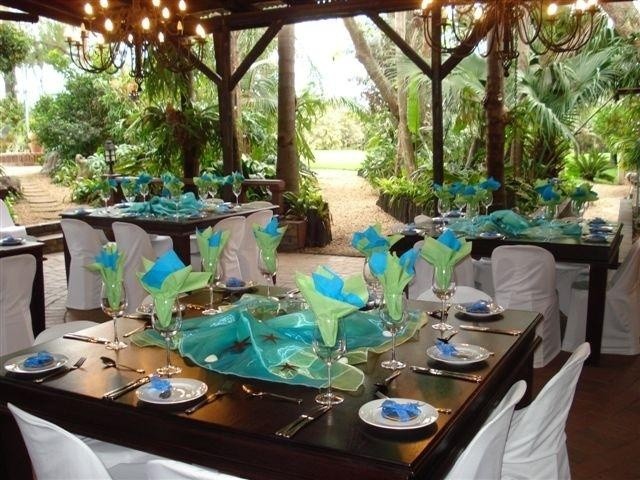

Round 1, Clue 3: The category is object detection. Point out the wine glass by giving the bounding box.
[481,191,494,217]
[100,279,128,350]
[363,254,384,310]
[100,187,112,215]
[436,197,451,230]
[454,193,466,221]
[198,188,208,215]
[257,248,280,303]
[139,184,150,202]
[379,292,409,370]
[314,313,347,406]
[208,185,217,205]
[231,183,243,208]
[151,298,184,374]
[170,190,184,219]
[431,265,457,330]
[543,204,559,241]
[200,257,223,315]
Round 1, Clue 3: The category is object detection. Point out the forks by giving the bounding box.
[34,356,87,384]
[185,378,234,415]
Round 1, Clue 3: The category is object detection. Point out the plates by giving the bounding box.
[123,212,141,218]
[426,343,490,367]
[135,376,207,405]
[1,237,23,246]
[400,228,423,236]
[187,214,204,220]
[479,232,502,238]
[116,203,131,208]
[357,397,439,430]
[585,233,609,242]
[3,351,68,373]
[454,301,504,319]
[136,300,187,315]
[591,225,612,233]
[75,208,91,216]
[216,280,260,290]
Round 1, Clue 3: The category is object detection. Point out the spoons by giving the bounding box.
[241,383,303,407]
[100,356,146,375]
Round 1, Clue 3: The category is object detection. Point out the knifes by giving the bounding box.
[103,373,161,402]
[412,365,481,386]
[461,325,522,338]
[64,333,111,346]
[275,404,331,440]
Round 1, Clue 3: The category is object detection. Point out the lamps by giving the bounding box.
[102,138,118,175]
[61,0,210,94]
[411,0,599,78]
